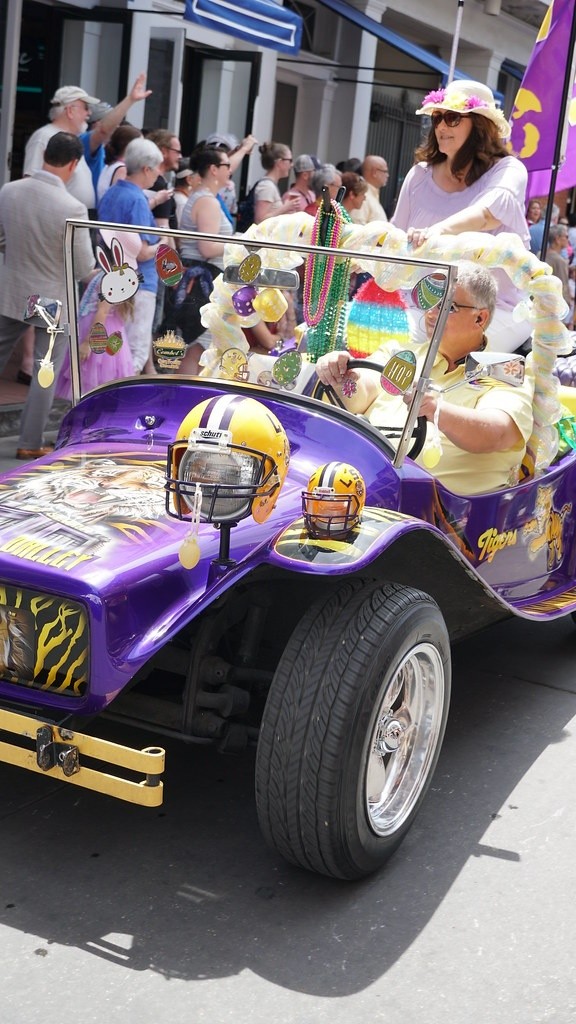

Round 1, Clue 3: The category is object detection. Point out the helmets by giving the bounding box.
[301,461,365,539]
[164,395,290,526]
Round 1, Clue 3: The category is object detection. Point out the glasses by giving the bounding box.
[209,163,230,170]
[358,177,364,183]
[431,110,471,127]
[274,158,292,164]
[559,235,568,240]
[163,146,181,154]
[64,105,88,111]
[438,300,481,313]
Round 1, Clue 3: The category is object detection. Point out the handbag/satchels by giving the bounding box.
[163,266,213,317]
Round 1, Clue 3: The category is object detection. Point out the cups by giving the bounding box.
[289,194,300,201]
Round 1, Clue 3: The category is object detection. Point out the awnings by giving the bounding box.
[318,0,505,108]
[95,0,303,55]
[502,64,524,81]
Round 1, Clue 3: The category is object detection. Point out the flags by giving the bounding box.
[502,0,576,210]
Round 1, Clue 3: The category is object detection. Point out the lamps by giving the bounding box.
[484,0,502,15]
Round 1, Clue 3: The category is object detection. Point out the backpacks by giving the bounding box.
[236,178,277,233]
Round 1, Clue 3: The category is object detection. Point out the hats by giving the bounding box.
[87,101,113,123]
[415,80,511,138]
[50,85,100,104]
[294,155,327,172]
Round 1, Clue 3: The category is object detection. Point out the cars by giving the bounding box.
[0,216,576,884]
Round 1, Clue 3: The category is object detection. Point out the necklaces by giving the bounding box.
[303,201,352,364]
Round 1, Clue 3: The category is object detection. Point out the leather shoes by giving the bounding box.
[16,447,54,460]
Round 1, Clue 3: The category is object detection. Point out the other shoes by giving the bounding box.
[17,370,33,385]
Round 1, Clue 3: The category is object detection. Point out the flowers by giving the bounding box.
[421,86,488,110]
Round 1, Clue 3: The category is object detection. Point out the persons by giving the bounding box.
[352,78,529,273]
[24,86,95,218]
[525,200,576,331]
[283,156,390,295]
[80,73,316,376]
[315,260,533,494]
[56,228,142,401]
[94,140,169,375]
[0,132,96,459]
